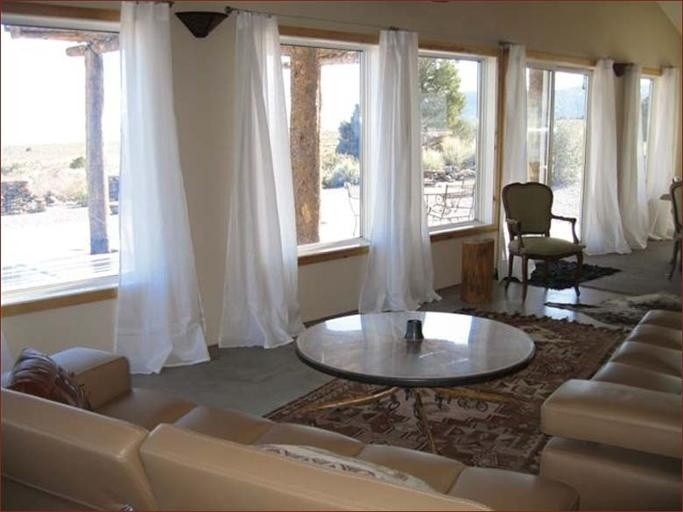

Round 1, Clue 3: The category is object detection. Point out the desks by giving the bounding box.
[293,310,540,454]
[659,192,678,265]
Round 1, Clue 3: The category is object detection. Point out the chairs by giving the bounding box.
[666,178,683,283]
[498,180,588,306]
[340,169,476,234]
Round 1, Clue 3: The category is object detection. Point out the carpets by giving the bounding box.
[542,289,681,327]
[260,305,628,478]
[491,253,626,293]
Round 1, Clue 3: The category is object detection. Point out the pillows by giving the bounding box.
[3,347,92,412]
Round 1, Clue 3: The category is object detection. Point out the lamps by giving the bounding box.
[612,61,633,77]
[173,10,227,41]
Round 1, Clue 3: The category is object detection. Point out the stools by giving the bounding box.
[459,237,495,304]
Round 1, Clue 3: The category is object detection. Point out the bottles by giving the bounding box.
[404,320,424,344]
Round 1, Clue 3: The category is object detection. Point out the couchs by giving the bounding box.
[0,344,578,510]
[540,298,683,510]
